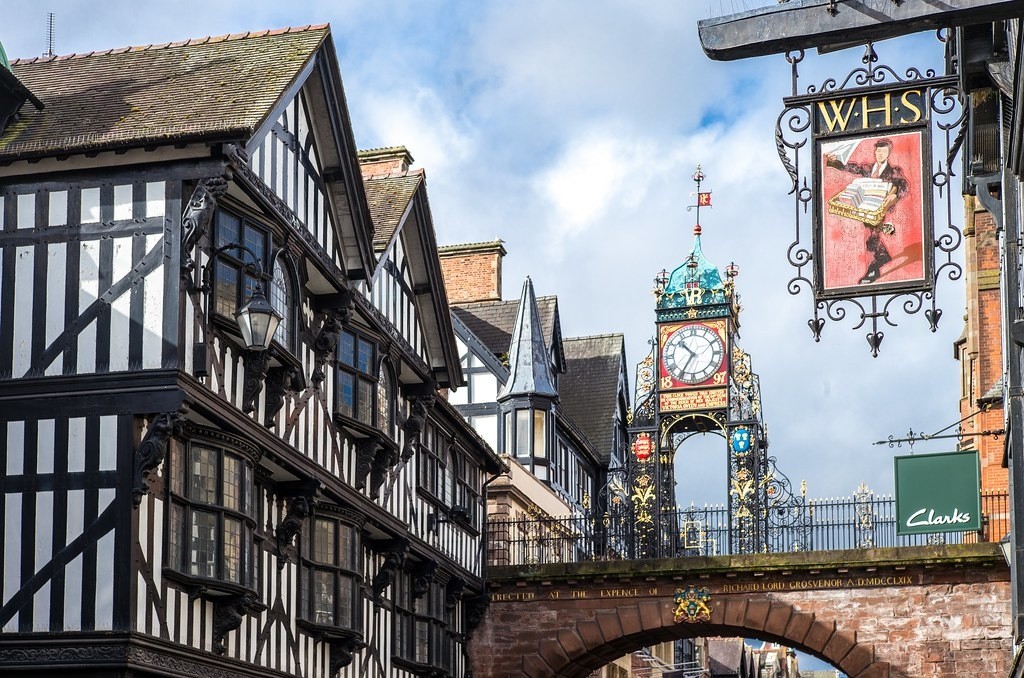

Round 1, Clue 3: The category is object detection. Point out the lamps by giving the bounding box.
[193,241,284,377]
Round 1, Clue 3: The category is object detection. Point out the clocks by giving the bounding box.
[663,324,725,383]
